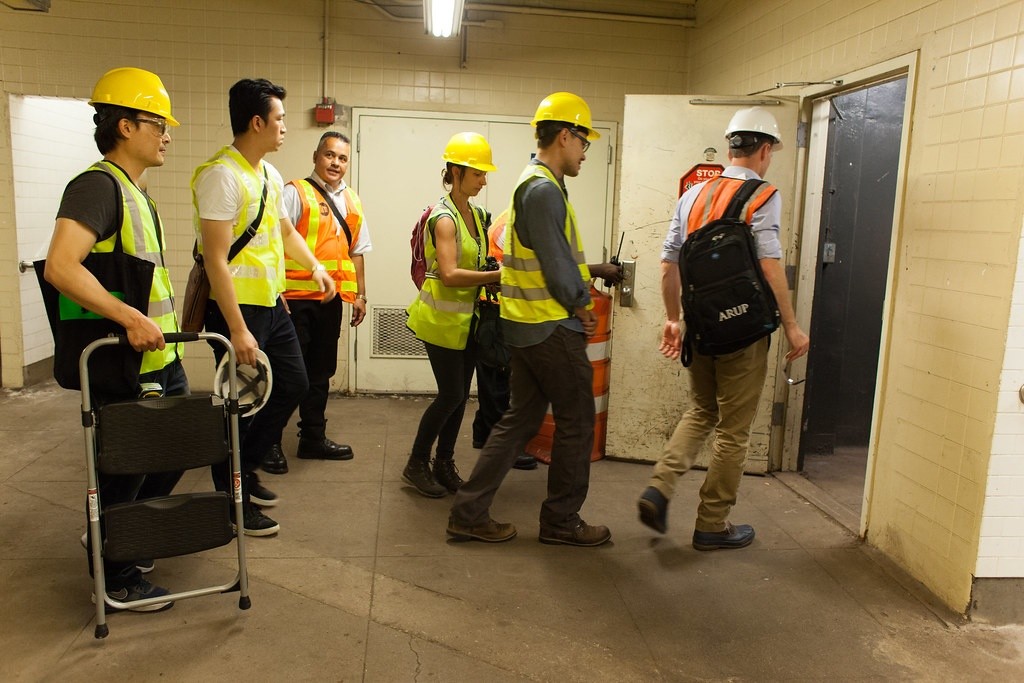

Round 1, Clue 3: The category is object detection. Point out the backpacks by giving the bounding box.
[411,196,487,291]
[679,178,782,368]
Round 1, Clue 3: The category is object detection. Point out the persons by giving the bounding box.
[191,77,337,537]
[44,67,191,613]
[401,131,501,498]
[637,106,809,552]
[256,132,372,476]
[446,91,611,547]
[472,209,537,468]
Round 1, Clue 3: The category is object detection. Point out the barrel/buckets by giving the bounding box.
[524,287,613,464]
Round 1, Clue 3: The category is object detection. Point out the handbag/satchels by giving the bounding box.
[181,255,210,332]
[33,170,155,401]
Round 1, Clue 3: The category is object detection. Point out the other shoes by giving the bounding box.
[472,432,488,449]
[512,451,537,469]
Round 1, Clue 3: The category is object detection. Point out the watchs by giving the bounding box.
[356,295,367,304]
[311,265,326,273]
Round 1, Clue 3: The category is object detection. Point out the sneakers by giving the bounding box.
[80,532,155,573]
[538,517,612,547]
[639,487,668,533]
[401,453,448,499]
[447,515,518,542]
[431,458,465,495]
[90,568,175,614]
[246,471,279,506]
[230,499,280,536]
[692,521,755,551]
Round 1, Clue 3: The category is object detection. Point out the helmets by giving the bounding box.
[531,92,600,141]
[88,67,180,127]
[214,347,273,417]
[441,132,497,172]
[725,105,783,152]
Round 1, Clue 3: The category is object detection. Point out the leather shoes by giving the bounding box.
[256,442,288,475]
[297,437,353,460]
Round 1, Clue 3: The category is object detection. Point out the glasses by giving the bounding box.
[782,349,808,386]
[558,126,591,153]
[115,115,169,137]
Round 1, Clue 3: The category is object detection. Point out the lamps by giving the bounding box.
[419,0,467,42]
